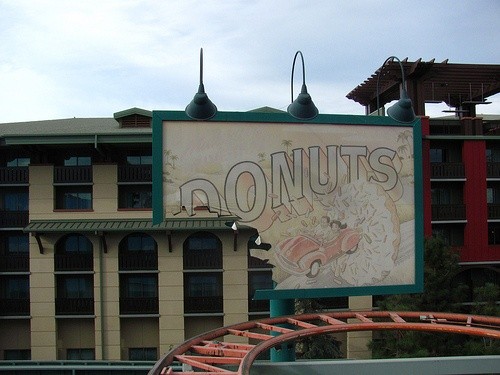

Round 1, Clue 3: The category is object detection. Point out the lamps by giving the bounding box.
[183,47,217,122]
[375,56,418,124]
[286,52,319,121]
